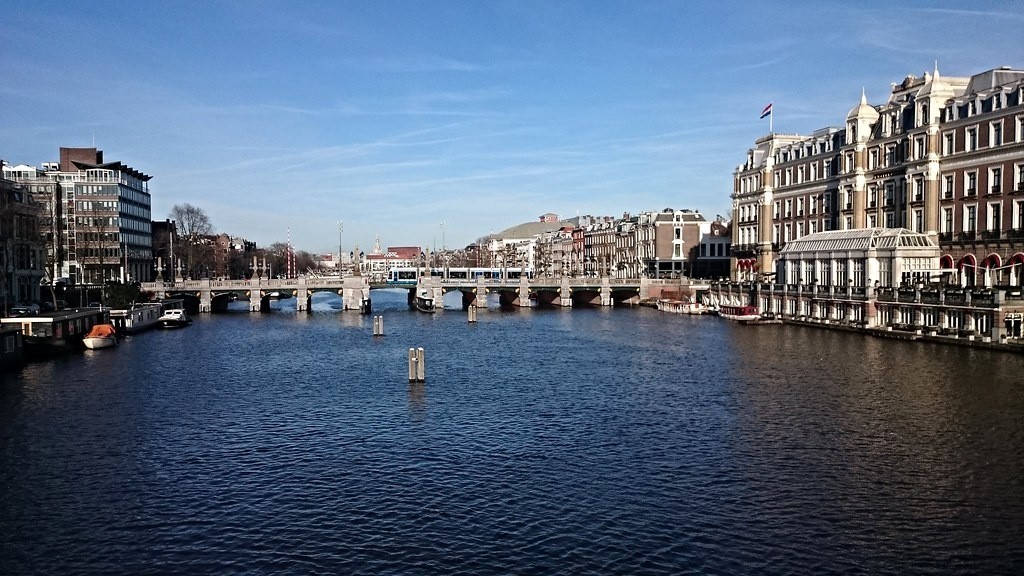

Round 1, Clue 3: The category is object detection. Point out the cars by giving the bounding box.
[0,299,71,318]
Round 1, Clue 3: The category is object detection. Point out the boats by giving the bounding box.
[83,324,117,348]
[655,298,711,315]
[717,304,762,320]
[158,309,193,328]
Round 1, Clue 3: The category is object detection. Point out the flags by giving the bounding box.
[760,103,772,119]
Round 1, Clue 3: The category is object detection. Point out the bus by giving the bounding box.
[385,267,535,284]
